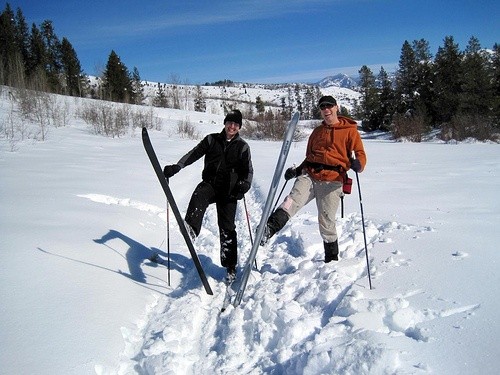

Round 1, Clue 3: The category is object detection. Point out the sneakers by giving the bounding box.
[180,219,197,243]
[225,265,238,281]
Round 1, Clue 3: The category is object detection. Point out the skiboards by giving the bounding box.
[142,127,237,313]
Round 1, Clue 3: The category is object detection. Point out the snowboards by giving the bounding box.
[232,112,301,309]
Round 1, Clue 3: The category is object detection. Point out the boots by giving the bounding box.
[324,239,338,262]
[256,209,289,246]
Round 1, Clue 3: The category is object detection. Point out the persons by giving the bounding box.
[164,110,253,283]
[257,96,366,263]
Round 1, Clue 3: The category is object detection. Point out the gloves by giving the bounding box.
[350,158,360,171]
[164,164,181,178]
[284,167,296,181]
[230,183,248,200]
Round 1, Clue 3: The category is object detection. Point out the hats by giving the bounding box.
[223,110,242,130]
[317,96,336,109]
